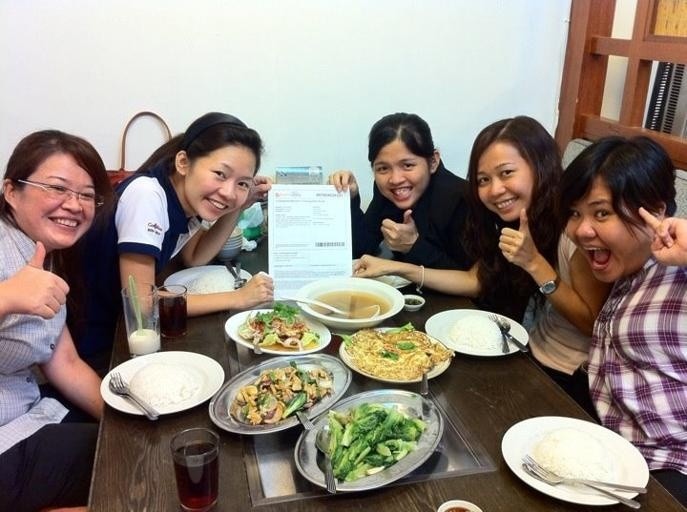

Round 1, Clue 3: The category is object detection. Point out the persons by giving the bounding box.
[353,117,613,424]
[329,113,474,271]
[559,135,687,508]
[0,130,104,512]
[64,113,275,378]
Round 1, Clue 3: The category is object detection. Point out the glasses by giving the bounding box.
[18,177,107,208]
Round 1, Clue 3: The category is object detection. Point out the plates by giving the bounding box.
[338,327,451,385]
[162,265,252,295]
[500,414,649,505]
[100,350,226,416]
[424,308,528,358]
[205,352,349,436]
[293,388,444,493]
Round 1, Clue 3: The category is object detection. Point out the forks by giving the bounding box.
[521,452,641,510]
[488,314,528,354]
[108,370,160,419]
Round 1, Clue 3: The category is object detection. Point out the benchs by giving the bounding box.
[559,138,687,227]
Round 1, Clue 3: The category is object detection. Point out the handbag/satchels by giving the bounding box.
[95,111,174,216]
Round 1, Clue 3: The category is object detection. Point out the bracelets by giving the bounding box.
[416,265,425,294]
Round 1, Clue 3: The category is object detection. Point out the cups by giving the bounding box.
[120,284,162,358]
[168,426,220,511]
[157,284,188,338]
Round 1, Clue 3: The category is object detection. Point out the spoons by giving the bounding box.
[521,459,648,495]
[498,315,510,354]
[316,423,340,495]
[225,261,244,289]
[272,293,380,317]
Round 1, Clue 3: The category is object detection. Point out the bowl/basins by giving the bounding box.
[215,227,243,262]
[295,276,405,331]
[436,498,483,512]
[400,293,425,312]
[223,307,332,355]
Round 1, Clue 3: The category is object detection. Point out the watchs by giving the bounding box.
[539,272,561,295]
